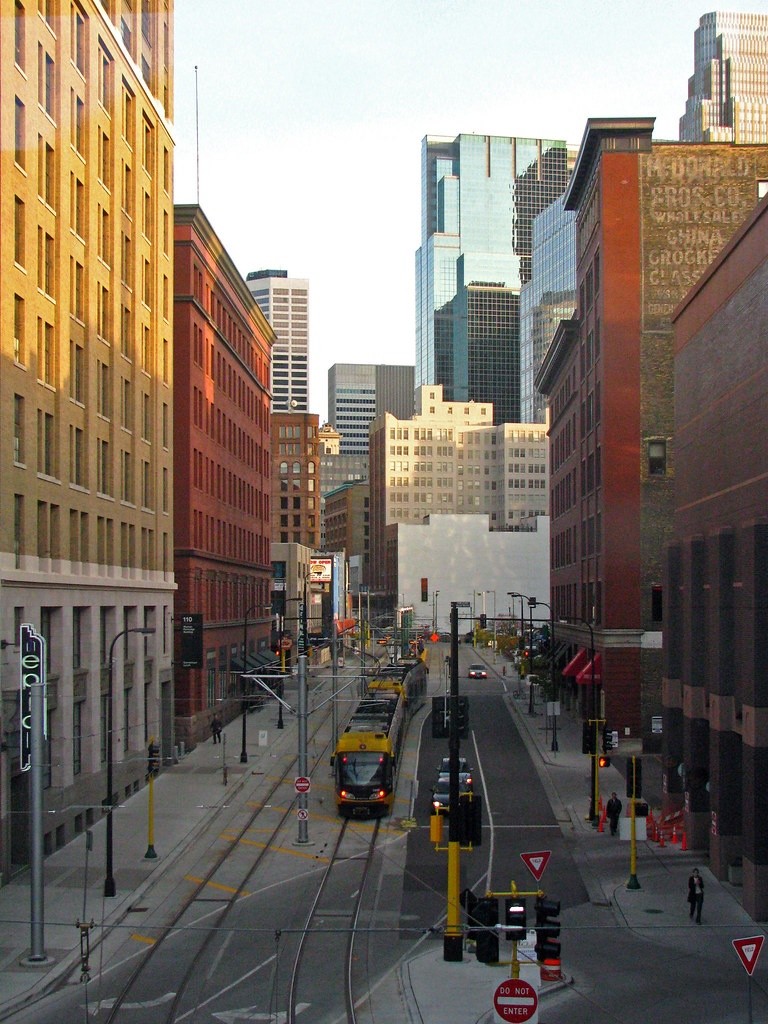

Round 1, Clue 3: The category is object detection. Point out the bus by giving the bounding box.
[329,647,431,822]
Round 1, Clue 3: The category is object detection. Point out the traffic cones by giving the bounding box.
[649,810,689,852]
[596,795,608,833]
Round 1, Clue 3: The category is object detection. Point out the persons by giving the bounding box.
[210,713,222,744]
[606,792,622,835]
[687,868,704,925]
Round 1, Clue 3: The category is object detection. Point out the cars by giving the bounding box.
[429,757,476,815]
[469,664,488,679]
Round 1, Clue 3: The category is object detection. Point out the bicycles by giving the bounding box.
[513,687,526,699]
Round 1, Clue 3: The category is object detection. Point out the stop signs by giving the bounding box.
[494,979,537,1022]
[295,777,311,793]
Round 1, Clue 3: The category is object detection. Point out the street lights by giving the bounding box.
[506,591,560,753]
[241,596,306,763]
[535,900,563,963]
[467,590,498,653]
[602,725,613,754]
[599,757,610,767]
[559,615,599,826]
[432,590,441,634]
[358,589,375,645]
[105,628,162,900]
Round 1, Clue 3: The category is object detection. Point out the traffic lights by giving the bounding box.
[150,743,161,775]
[519,637,525,650]
